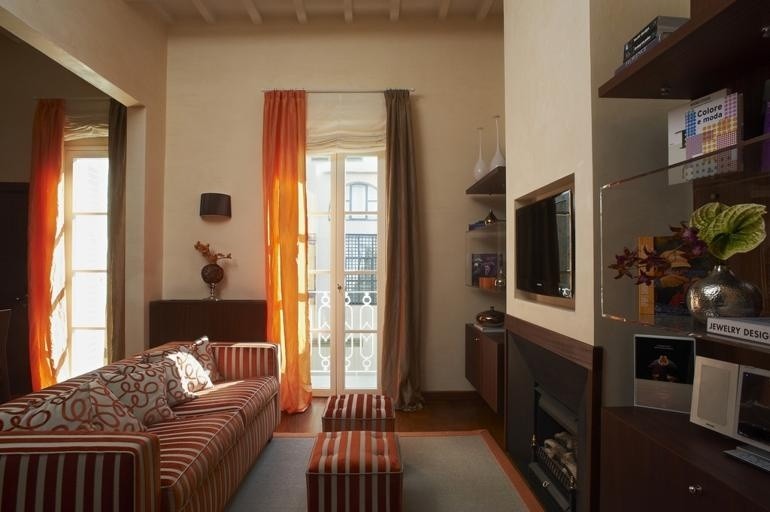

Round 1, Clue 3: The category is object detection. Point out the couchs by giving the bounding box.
[0,340,280,512]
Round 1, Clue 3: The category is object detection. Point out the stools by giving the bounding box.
[305,431,403,512]
[322,393,396,431]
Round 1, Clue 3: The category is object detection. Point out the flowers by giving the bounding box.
[193,240,232,263]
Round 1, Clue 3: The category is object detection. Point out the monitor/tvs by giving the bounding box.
[515,187,574,300]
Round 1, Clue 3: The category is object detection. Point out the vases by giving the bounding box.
[201,263,223,301]
[488,115,505,171]
[472,126,488,183]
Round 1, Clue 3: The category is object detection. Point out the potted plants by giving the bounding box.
[608,202,767,328]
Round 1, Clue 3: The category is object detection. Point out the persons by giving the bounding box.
[657,248,724,313]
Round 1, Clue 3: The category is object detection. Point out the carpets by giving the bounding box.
[224,432,549,512]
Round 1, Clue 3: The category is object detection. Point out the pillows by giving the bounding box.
[0,335,223,433]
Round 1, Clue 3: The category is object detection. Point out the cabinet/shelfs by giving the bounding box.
[594,2,770,512]
[463,165,505,415]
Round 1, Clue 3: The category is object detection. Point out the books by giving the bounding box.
[637,231,723,317]
[470,253,498,286]
[665,85,732,185]
[613,31,673,76]
[682,92,744,182]
[623,25,680,63]
[621,16,692,53]
